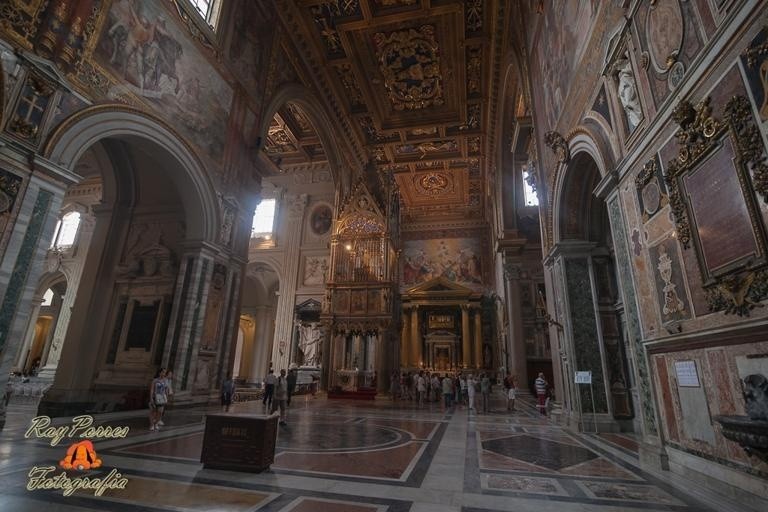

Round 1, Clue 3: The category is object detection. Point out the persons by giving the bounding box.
[150,368,173,430]
[534,373,548,413]
[263,366,296,425]
[218,370,236,413]
[404,250,483,285]
[390,369,515,412]
[107,1,175,94]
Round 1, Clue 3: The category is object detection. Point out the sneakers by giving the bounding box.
[150,420,164,430]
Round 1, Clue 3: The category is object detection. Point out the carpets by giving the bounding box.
[328,387,377,401]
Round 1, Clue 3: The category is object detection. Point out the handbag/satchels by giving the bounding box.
[155,393,167,405]
